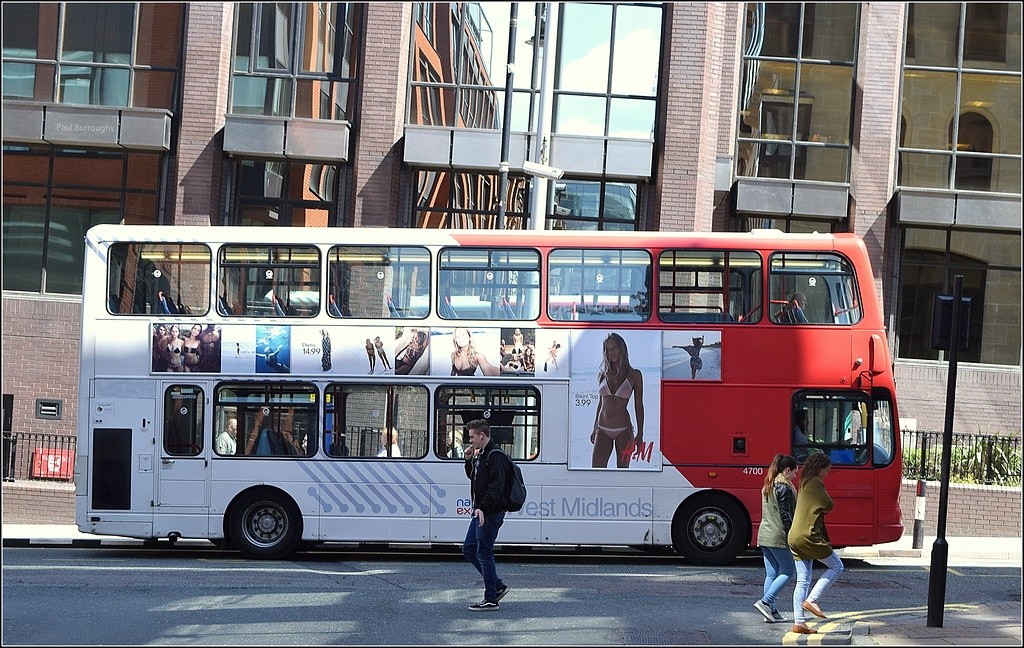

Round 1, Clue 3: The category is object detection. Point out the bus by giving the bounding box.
[75,228,903,562]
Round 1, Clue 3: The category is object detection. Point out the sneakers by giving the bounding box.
[764,613,787,621]
[754,600,776,622]
[496,585,509,601]
[469,600,500,611]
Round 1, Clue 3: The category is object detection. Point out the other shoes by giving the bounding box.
[793,626,817,634]
[801,601,827,619]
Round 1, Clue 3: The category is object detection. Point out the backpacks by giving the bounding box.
[486,449,527,512]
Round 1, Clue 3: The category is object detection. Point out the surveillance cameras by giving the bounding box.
[522,161,564,179]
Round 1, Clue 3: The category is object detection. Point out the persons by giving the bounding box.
[787,453,845,634]
[672,335,704,379]
[754,453,798,623]
[152,324,221,372]
[377,428,401,457]
[447,429,464,458]
[255,327,561,377]
[793,409,839,467]
[464,419,509,612]
[215,418,237,455]
[590,333,645,468]
[791,292,807,311]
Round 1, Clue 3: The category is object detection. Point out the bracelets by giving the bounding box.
[830,442,833,448]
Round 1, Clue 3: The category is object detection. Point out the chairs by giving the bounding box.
[158,293,519,319]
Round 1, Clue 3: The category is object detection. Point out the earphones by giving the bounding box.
[481,435,483,439]
